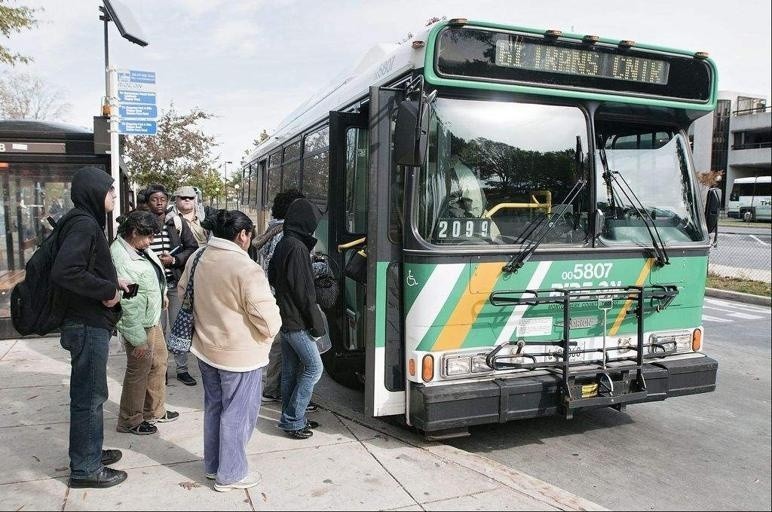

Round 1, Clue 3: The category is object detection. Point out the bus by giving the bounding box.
[232,16,726,444]
[727,176,771,224]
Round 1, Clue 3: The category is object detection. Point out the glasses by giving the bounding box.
[180,196,195,200]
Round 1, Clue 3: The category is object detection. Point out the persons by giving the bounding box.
[12,196,65,246]
[56,167,138,487]
[253,190,320,413]
[109,209,181,437]
[137,184,220,387]
[385,135,487,391]
[268,199,327,439]
[177,208,282,491]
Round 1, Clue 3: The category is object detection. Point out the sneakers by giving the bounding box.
[130,420,158,436]
[158,411,180,422]
[205,469,263,492]
[176,372,197,386]
[262,393,282,402]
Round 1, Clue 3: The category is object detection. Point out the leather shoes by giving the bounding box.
[68,466,128,489]
[102,449,123,465]
[283,402,319,440]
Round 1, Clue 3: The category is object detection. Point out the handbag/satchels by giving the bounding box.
[310,254,342,310]
[344,249,366,287]
[166,284,196,354]
[433,191,478,245]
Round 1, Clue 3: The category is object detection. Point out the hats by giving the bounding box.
[171,185,197,198]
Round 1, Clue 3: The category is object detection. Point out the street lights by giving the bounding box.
[224,160,232,208]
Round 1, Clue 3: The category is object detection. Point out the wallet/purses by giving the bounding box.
[123,283,139,299]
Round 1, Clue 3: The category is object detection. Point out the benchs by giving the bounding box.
[0,265,28,319]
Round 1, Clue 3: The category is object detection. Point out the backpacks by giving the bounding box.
[10,212,101,338]
